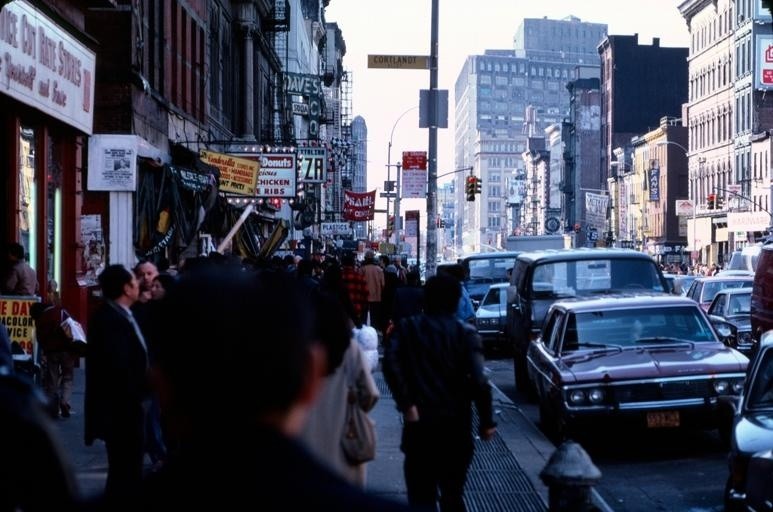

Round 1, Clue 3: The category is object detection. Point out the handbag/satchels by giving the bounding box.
[340,385,377,468]
[61,309,88,357]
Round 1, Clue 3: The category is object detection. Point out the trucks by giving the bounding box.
[751,243,772,339]
[727,246,762,273]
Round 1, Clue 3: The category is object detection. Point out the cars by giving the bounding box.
[714,270,755,289]
[526,291,750,432]
[406,236,565,344]
[685,276,754,314]
[725,329,773,512]
[706,288,752,354]
[663,273,700,297]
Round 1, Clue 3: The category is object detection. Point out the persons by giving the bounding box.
[6,215,516,508]
[647,246,724,277]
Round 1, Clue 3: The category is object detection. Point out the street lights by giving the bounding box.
[656,141,697,267]
[609,160,645,251]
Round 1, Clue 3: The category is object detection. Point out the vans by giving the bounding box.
[505,247,681,391]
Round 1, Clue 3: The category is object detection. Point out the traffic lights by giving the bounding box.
[465,175,476,202]
[386,215,394,237]
[439,219,445,229]
[709,194,714,209]
[715,194,723,210]
[706,195,709,209]
[575,223,579,234]
[475,178,481,194]
